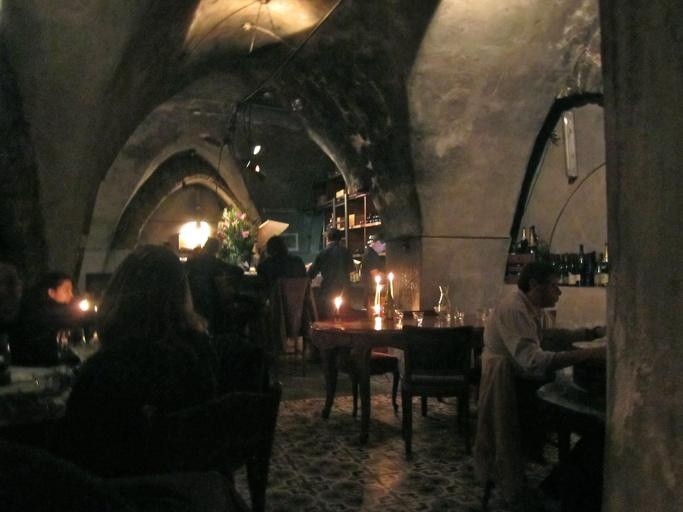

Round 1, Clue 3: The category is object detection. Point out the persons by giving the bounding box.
[482,263,607,428]
[255,236,305,279]
[360,231,386,287]
[308,228,355,320]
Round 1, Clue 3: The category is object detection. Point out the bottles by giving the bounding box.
[437,284,451,312]
[510,225,609,287]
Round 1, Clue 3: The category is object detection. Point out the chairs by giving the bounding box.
[481,431,570,512]
[310,286,482,455]
[142,382,281,512]
[265,277,317,377]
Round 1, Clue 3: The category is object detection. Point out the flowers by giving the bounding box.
[216,209,258,268]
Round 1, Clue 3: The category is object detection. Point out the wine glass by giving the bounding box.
[67,323,99,361]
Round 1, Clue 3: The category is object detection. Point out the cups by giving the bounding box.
[411,312,425,319]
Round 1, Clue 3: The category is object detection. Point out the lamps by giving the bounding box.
[258,219,289,247]
[231,102,261,172]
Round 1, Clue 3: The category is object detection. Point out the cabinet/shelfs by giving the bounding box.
[314,191,381,260]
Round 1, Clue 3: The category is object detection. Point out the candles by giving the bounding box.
[374,277,380,311]
[389,273,394,297]
